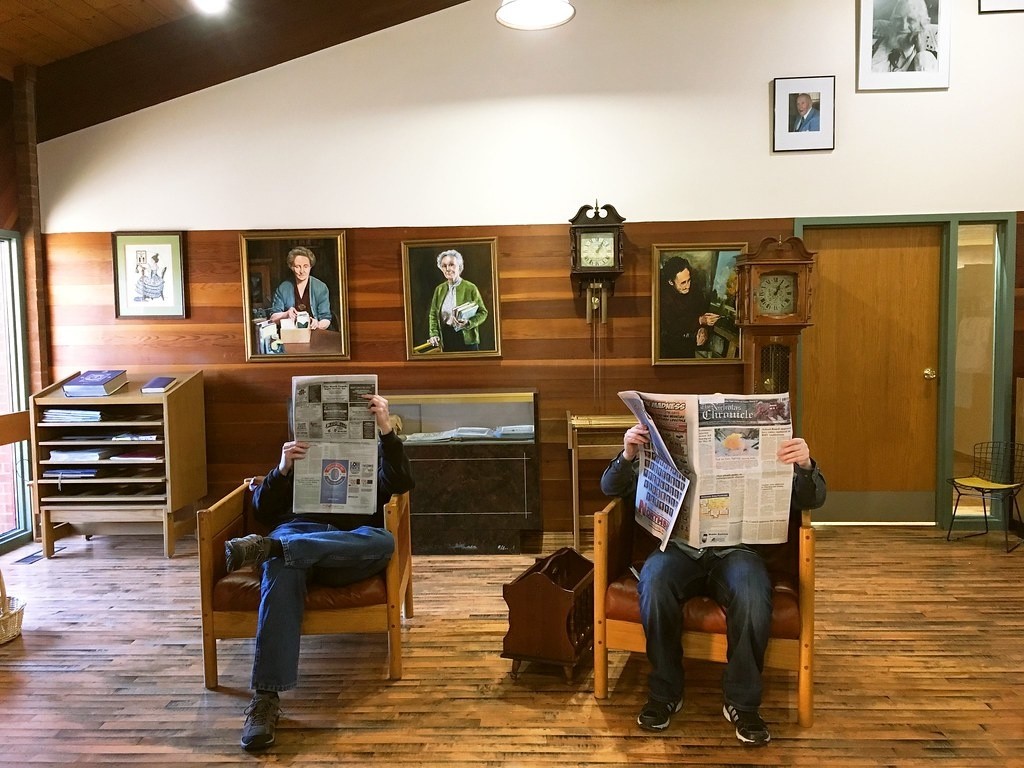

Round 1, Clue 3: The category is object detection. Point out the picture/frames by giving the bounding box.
[401,237,502,361]
[238,230,350,362]
[111,230,186,319]
[651,242,748,367]
[977,0,1024,15]
[855,0,952,94]
[773,75,836,153]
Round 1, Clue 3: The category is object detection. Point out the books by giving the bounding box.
[42,369,179,479]
[252,318,280,354]
[452,302,479,332]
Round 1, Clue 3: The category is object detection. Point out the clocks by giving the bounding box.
[733,235,817,447]
[568,196,626,399]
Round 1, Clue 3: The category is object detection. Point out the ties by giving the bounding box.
[795,117,804,132]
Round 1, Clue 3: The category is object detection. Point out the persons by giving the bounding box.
[792,93,820,132]
[660,256,721,358]
[644,444,685,517]
[872,0,938,72]
[270,335,287,353]
[270,246,332,330]
[601,424,828,746]
[224,394,416,749]
[427,250,488,352]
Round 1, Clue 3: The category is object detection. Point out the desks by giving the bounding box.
[567,410,640,553]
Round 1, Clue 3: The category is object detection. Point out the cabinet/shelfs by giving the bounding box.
[367,387,543,555]
[29,370,207,559]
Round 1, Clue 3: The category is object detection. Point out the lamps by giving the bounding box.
[495,0,576,31]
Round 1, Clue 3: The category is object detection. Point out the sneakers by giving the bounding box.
[224,534,265,574]
[722,703,771,746]
[637,694,684,731]
[241,695,285,751]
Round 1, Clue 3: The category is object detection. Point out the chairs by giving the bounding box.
[946,441,1024,553]
[594,493,816,727]
[196,482,413,688]
